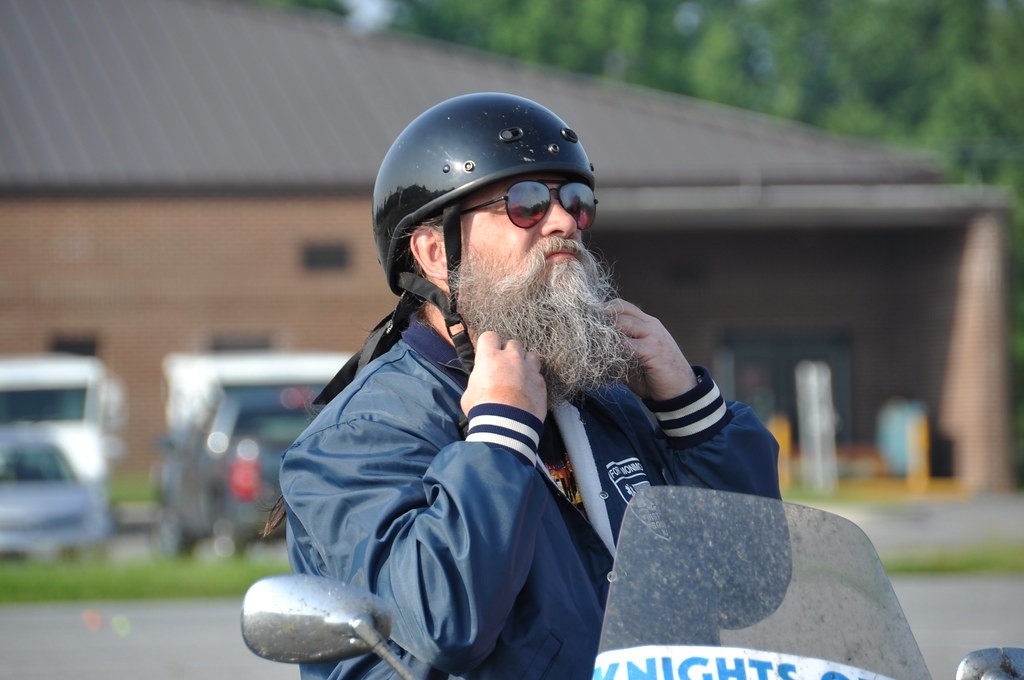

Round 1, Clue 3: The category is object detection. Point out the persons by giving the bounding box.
[278,92,791,680]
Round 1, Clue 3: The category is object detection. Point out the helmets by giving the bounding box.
[372,92,595,298]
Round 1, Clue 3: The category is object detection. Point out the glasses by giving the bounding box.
[435,180,599,230]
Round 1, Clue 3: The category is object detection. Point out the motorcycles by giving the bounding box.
[241,485,1024,680]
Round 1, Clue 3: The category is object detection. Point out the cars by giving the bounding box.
[160,386,334,555]
[0,441,105,554]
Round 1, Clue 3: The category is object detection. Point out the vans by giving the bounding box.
[0,354,126,479]
[162,350,354,433]
